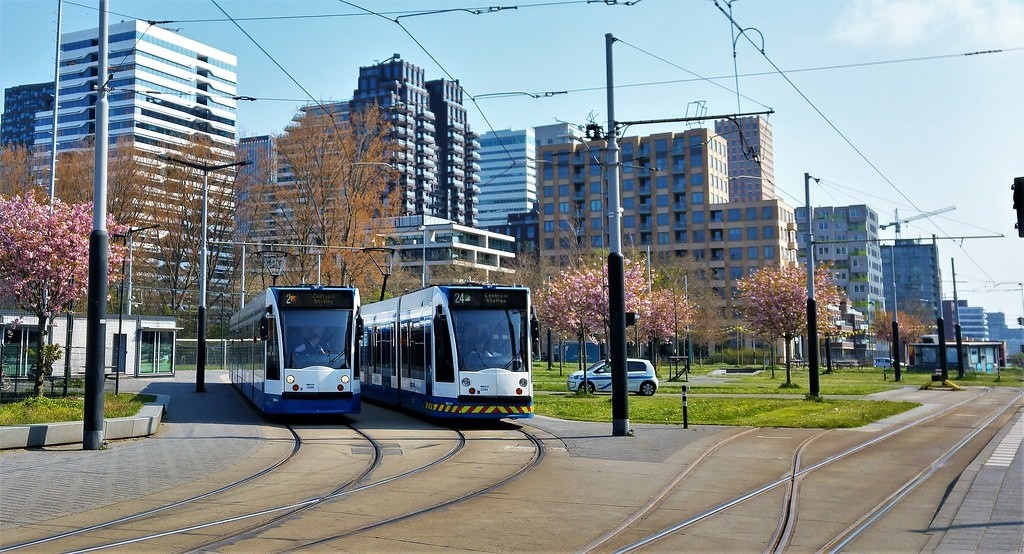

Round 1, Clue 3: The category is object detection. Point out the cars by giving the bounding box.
[567,357,659,397]
[872,357,908,367]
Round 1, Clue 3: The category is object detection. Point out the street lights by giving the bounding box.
[156,154,254,393]
[111,222,161,316]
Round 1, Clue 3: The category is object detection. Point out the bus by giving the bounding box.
[226,250,365,416]
[358,247,540,425]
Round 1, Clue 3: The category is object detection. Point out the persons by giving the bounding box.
[601,362,611,373]
[459,322,487,356]
[294,334,327,357]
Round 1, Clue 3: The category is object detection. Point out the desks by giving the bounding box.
[79,366,118,382]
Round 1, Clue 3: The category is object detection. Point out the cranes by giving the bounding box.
[878,205,957,240]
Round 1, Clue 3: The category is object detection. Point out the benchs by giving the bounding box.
[78,371,124,376]
